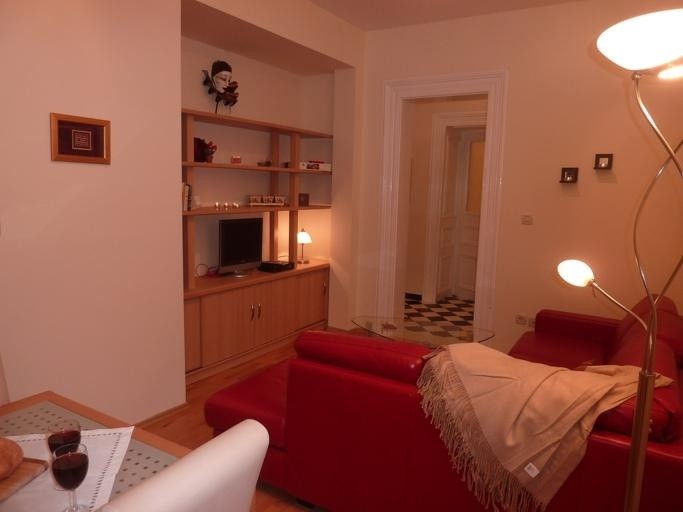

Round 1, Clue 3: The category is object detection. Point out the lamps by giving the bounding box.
[557,8,683,511]
[297,228,312,264]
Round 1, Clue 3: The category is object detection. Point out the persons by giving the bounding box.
[211,61,233,94]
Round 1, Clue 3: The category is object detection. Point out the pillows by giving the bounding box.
[292,327,429,382]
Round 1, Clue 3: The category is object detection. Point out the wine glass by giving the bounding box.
[45,418,89,512]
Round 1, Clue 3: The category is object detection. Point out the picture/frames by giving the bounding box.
[49,113,111,165]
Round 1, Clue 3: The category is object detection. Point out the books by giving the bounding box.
[296,160,331,172]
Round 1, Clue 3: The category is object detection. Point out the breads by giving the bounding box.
[0,438,23,481]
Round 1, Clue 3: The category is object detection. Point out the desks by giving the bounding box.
[1,390,190,512]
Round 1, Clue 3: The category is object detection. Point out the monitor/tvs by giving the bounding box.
[217,217,263,277]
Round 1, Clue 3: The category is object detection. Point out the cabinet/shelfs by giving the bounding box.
[183,107,334,378]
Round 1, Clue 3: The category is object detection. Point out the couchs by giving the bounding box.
[205,293,683,512]
